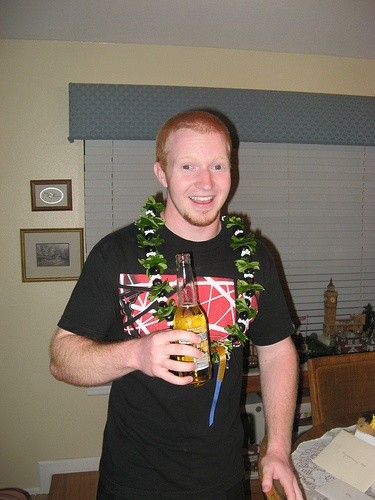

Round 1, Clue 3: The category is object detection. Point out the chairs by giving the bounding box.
[307,352,375,427]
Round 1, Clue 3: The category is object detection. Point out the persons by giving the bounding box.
[50,110,304,500]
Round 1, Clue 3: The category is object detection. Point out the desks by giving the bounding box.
[247,350,310,393]
[287,412,375,500]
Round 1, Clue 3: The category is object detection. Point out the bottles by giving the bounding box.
[172,253,213,386]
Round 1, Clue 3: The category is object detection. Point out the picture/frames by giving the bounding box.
[30,179,73,212]
[20,228,84,282]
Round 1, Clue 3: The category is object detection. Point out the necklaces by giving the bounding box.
[137,195,264,364]
